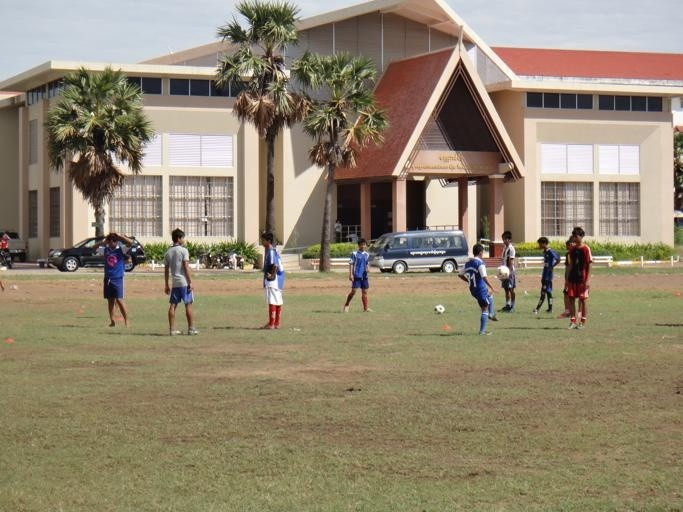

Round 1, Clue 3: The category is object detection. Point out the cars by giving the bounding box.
[47,234,147,273]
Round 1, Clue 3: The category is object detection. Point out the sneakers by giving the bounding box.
[498,305,511,313]
[188,329,199,335]
[275,324,280,327]
[344,305,349,312]
[170,329,183,336]
[364,307,372,312]
[567,318,577,330]
[479,331,491,336]
[265,324,274,328]
[489,312,499,321]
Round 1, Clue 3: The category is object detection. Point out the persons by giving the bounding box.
[164,229,199,335]
[556,236,576,318]
[334,219,342,243]
[458,244,498,335]
[532,237,560,314]
[261,233,284,330]
[501,229,515,312]
[92,233,133,327]
[344,239,371,314]
[565,227,592,329]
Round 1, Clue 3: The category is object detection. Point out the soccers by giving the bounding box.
[434,304,445,314]
[497,265,510,280]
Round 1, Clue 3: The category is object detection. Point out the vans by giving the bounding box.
[368,228,473,277]
